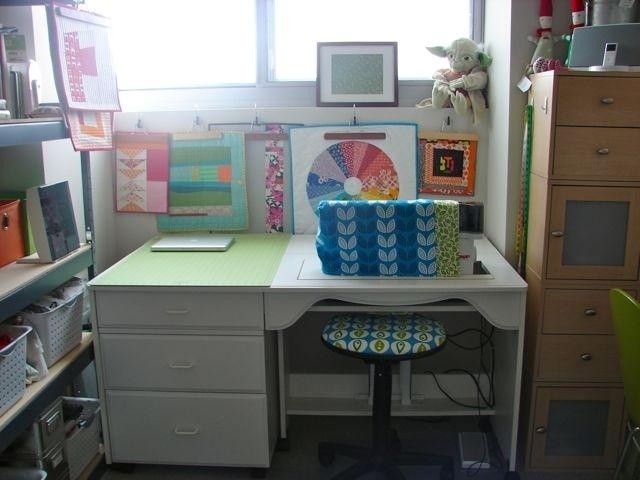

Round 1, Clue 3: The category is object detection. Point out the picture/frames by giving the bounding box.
[315,40,399,108]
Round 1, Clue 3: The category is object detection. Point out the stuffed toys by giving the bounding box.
[562,0,585,65]
[525,0,571,75]
[425,38,494,125]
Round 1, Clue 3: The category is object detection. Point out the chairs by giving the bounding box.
[608,287,640,479]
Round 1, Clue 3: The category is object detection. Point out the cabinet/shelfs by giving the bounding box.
[523,67,640,476]
[0,0,98,480]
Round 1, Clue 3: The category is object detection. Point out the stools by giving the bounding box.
[316,314,456,479]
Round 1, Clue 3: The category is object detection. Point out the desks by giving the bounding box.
[84,230,528,480]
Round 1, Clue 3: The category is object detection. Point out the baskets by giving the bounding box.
[0,467,48,480]
[60,396,103,480]
[0,325,33,418]
[17,287,86,370]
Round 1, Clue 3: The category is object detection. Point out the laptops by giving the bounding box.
[150,235,236,252]
